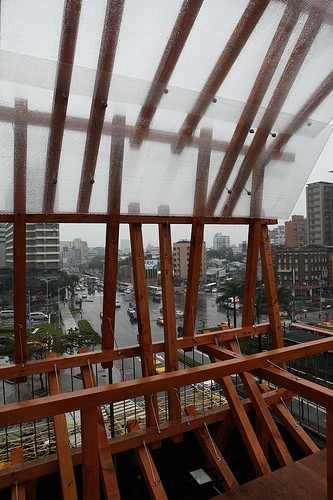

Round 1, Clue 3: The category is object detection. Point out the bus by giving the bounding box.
[204,282,217,292]
[218,295,240,309]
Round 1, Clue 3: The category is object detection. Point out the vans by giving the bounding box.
[0,309,14,319]
[28,311,49,321]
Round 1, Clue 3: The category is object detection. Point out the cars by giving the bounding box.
[115,299,121,308]
[156,317,164,325]
[176,310,184,316]
[320,321,333,330]
[153,359,165,374]
[217,322,234,329]
[73,275,162,302]
[127,308,136,316]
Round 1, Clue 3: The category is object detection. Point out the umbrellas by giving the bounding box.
[303,310,307,312]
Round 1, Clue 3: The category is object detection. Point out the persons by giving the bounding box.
[305,311,307,318]
